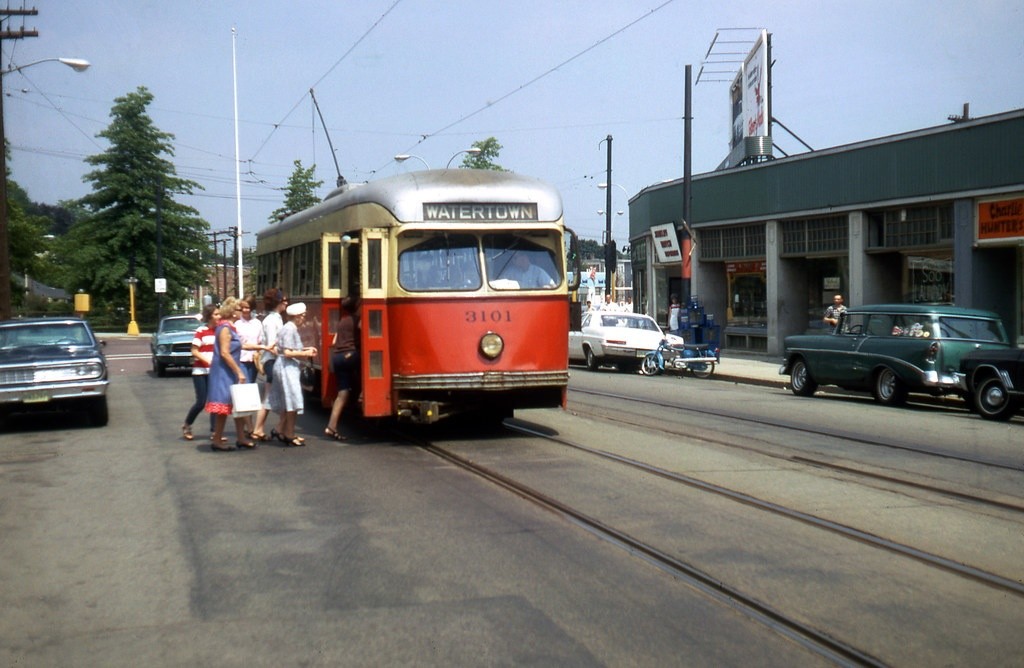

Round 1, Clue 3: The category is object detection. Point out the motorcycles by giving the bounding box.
[640,331,721,379]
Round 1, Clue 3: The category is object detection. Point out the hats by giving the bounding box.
[286,302,307,316]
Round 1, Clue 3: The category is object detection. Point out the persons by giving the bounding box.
[323,297,362,440]
[586,301,595,311]
[625,296,633,313]
[823,294,849,333]
[181,288,317,449]
[602,294,620,312]
[668,294,681,331]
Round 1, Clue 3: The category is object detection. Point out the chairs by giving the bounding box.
[868,318,894,336]
[603,318,616,327]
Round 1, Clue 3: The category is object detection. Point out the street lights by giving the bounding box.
[1,57,90,320]
[597,183,631,200]
[394,147,482,171]
[598,210,624,240]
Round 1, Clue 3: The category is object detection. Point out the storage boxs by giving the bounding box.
[668,307,721,345]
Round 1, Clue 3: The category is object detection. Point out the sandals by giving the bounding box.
[270,429,285,442]
[324,428,343,441]
[249,432,271,442]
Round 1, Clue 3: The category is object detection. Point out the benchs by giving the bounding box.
[9,345,71,355]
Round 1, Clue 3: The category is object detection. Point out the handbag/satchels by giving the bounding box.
[228,382,263,419]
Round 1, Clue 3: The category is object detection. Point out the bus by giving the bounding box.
[253,168,583,427]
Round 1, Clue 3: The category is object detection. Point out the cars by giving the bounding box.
[149,315,203,374]
[952,347,1024,421]
[0,316,110,416]
[778,305,1012,406]
[568,311,673,373]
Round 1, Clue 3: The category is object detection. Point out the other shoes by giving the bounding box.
[210,432,228,440]
[244,429,254,436]
[182,425,194,440]
[236,440,257,449]
[211,442,233,452]
[282,435,305,446]
